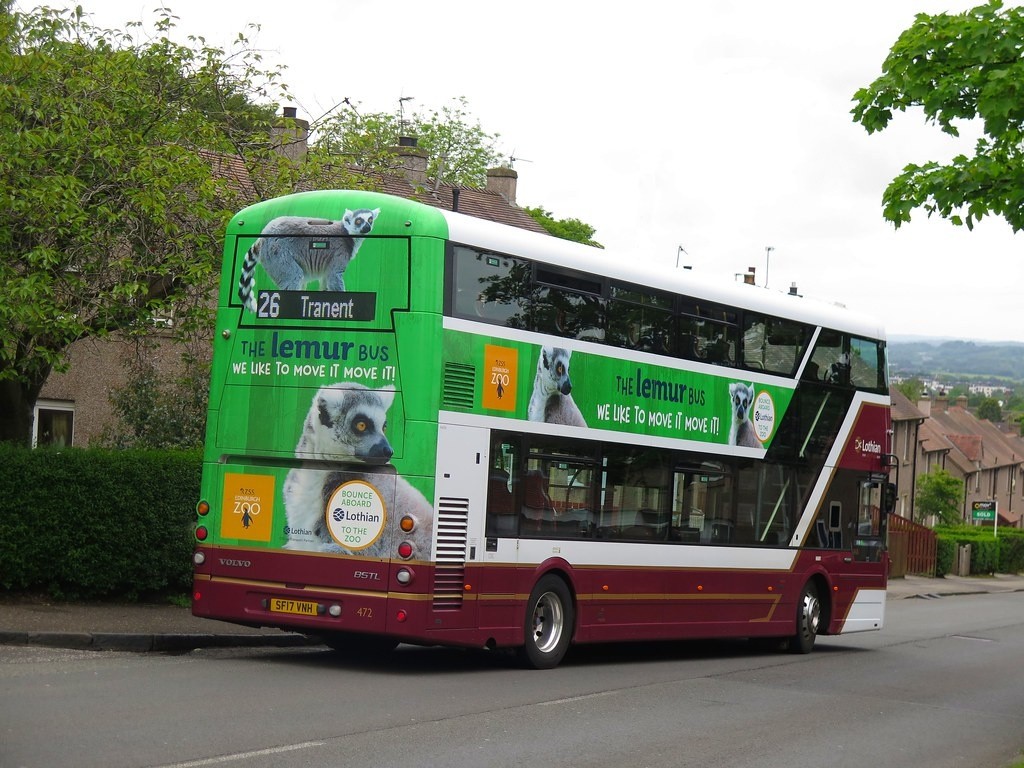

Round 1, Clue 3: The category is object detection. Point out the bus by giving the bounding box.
[191,187,900,686]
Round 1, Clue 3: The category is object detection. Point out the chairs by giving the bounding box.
[494,468,742,543]
